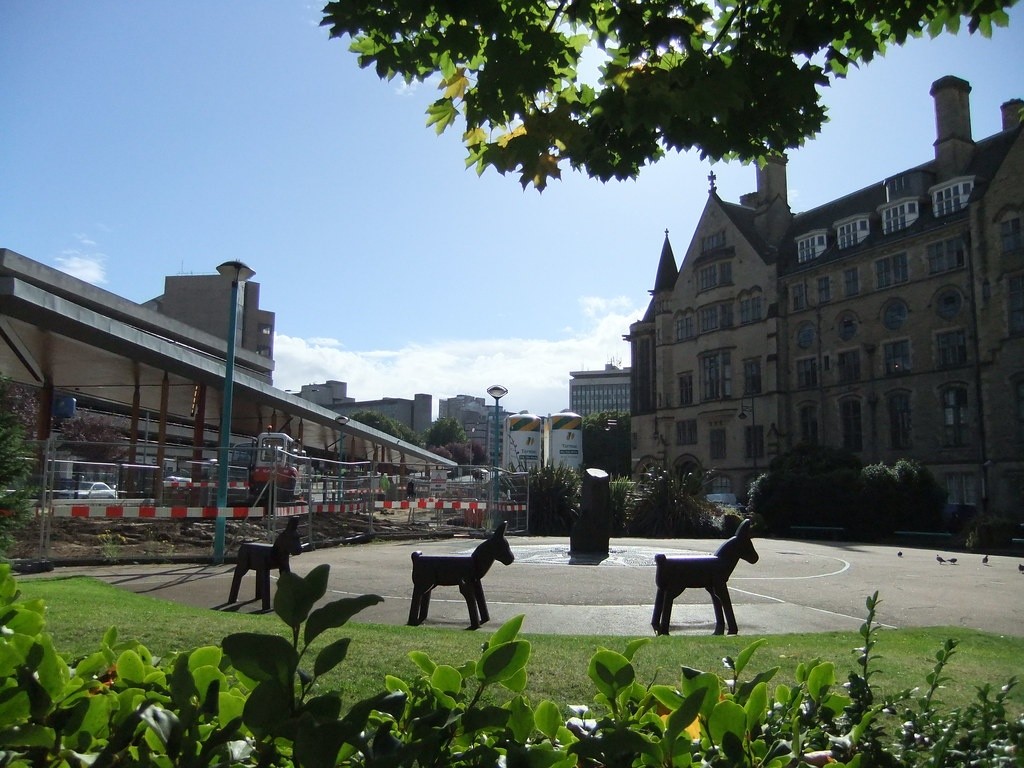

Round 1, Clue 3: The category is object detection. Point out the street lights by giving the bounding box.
[487,385,509,530]
[212,261,258,563]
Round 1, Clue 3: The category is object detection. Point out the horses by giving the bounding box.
[652,518,758,635]
[407,520,515,628]
[227,516,303,610]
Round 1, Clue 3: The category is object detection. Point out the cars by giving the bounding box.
[48,480,117,499]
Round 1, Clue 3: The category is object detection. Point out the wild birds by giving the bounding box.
[898,551,902,557]
[936,554,946,565]
[1017,564,1024,575]
[948,558,957,564]
[982,555,989,564]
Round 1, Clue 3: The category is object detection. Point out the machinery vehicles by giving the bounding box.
[208,423,316,507]
